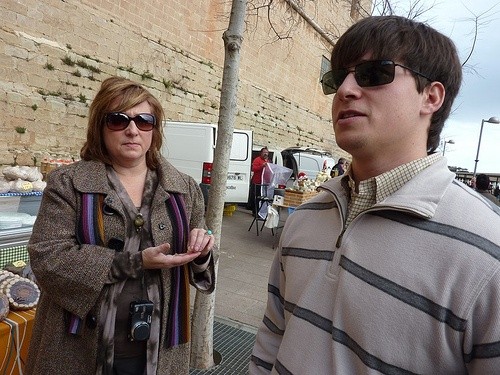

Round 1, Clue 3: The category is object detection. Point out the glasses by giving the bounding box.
[106,111,157,132]
[320,58,432,97]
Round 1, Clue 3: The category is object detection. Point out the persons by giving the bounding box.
[27,77,220,375]
[247,17,499,374]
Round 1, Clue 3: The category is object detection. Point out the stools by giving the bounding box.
[247,183,274,237]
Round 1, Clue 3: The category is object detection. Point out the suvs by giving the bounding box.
[251,138,332,191]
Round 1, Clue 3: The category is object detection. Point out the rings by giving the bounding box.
[205,230,212,236]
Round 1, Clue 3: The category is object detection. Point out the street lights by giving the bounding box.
[443,138,455,156]
[471,116,500,190]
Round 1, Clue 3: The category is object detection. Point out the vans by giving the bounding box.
[293,152,335,182]
[162,120,254,209]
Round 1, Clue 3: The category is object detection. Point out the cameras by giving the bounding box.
[128,299,154,341]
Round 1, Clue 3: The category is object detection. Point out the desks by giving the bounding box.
[272,203,297,242]
[0,307,36,375]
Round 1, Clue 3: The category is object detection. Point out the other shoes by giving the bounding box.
[252,213,260,218]
